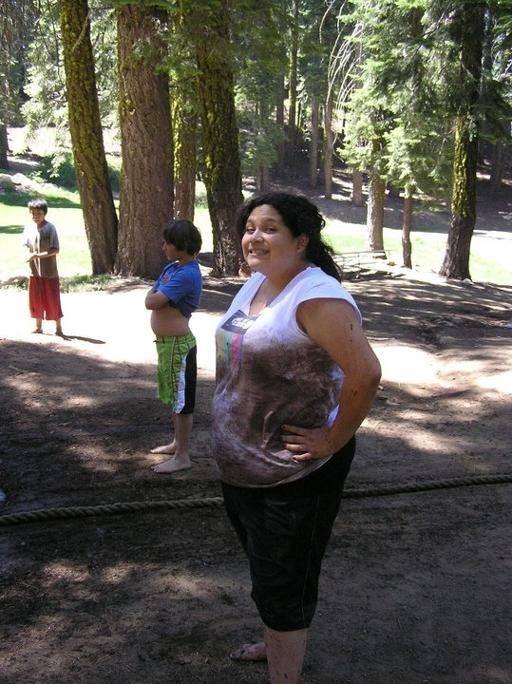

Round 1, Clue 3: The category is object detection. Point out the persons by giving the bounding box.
[23,199,63,336]
[211,193,381,684]
[145,220,202,473]
